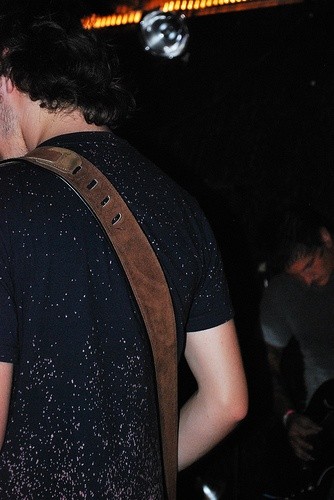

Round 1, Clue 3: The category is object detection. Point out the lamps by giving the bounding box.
[139,10,190,60]
